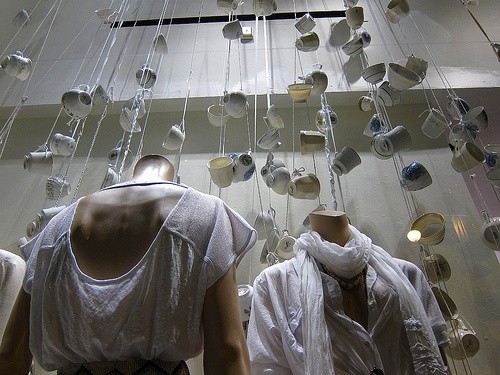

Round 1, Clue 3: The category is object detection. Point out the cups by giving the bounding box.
[302,204,326,230]
[236,284,254,322]
[432,286,457,318]
[206,0,362,201]
[358,57,429,112]
[410,213,445,246]
[385,0,410,25]
[2,50,32,80]
[11,9,32,28]
[450,140,486,173]
[94,8,118,24]
[353,30,371,48]
[445,317,481,361]
[253,208,298,265]
[480,211,500,251]
[420,109,453,139]
[362,114,412,160]
[400,160,432,192]
[448,124,476,148]
[345,7,364,30]
[153,32,168,55]
[424,252,451,285]
[342,36,364,56]
[23,62,186,239]
[461,106,489,133]
[483,144,500,186]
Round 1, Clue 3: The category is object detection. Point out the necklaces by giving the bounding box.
[323,265,384,375]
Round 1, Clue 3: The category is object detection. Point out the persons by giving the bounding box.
[247,211,451,375]
[0,155,251,375]
